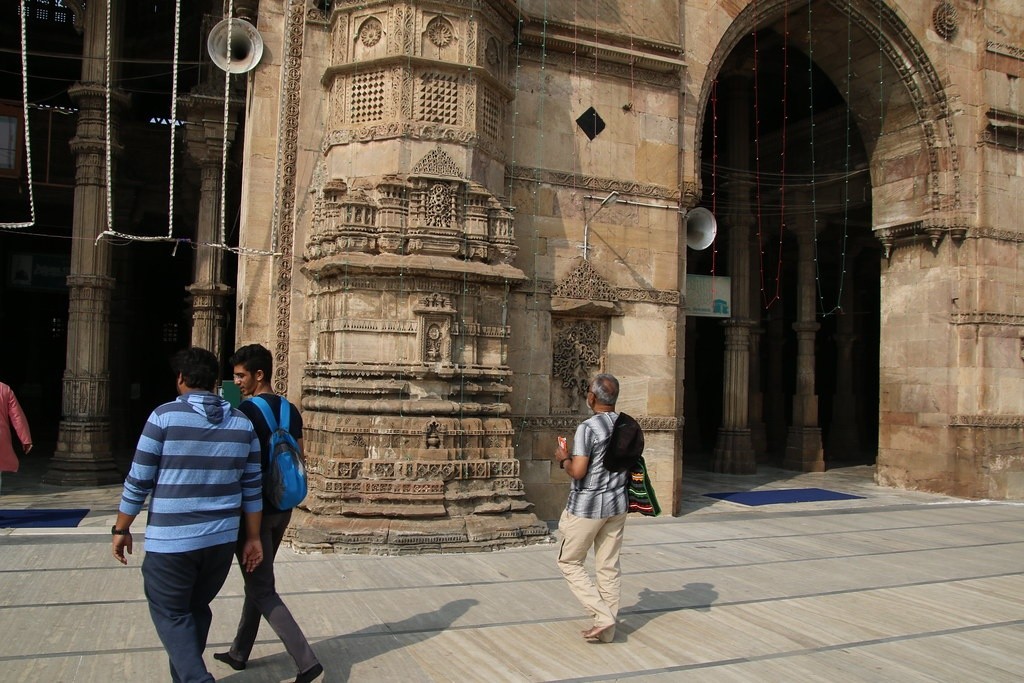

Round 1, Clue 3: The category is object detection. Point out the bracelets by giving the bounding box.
[560,458,572,469]
[112,525,129,535]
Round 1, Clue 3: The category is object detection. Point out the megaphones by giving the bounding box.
[685,207,717,251]
[207,19,264,73]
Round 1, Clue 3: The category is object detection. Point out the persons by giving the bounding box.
[554,374,629,644]
[214,344,323,683]
[112,347,263,683]
[0,381,33,490]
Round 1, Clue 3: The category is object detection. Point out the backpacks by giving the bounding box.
[241,395,308,511]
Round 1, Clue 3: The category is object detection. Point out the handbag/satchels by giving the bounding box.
[626,457,662,516]
